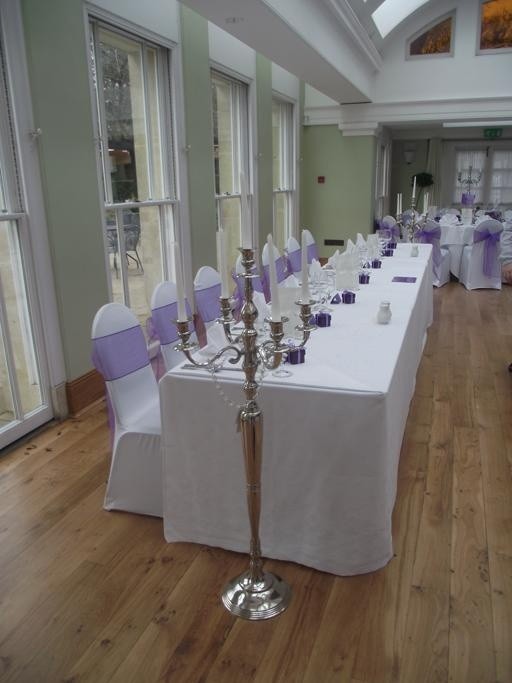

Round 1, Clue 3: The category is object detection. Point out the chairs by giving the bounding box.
[381,206,512,291]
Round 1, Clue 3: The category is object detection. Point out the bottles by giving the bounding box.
[411,245,419,257]
[378,300,391,324]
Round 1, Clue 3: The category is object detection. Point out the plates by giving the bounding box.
[456,222,463,226]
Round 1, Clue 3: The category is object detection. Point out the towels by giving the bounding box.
[330,233,380,271]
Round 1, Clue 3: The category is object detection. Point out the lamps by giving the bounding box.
[457,165,484,194]
[173,230,316,621]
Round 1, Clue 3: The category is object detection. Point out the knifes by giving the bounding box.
[182,364,242,373]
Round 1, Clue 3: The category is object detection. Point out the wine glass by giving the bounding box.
[357,246,369,274]
[376,229,392,255]
[312,270,328,306]
